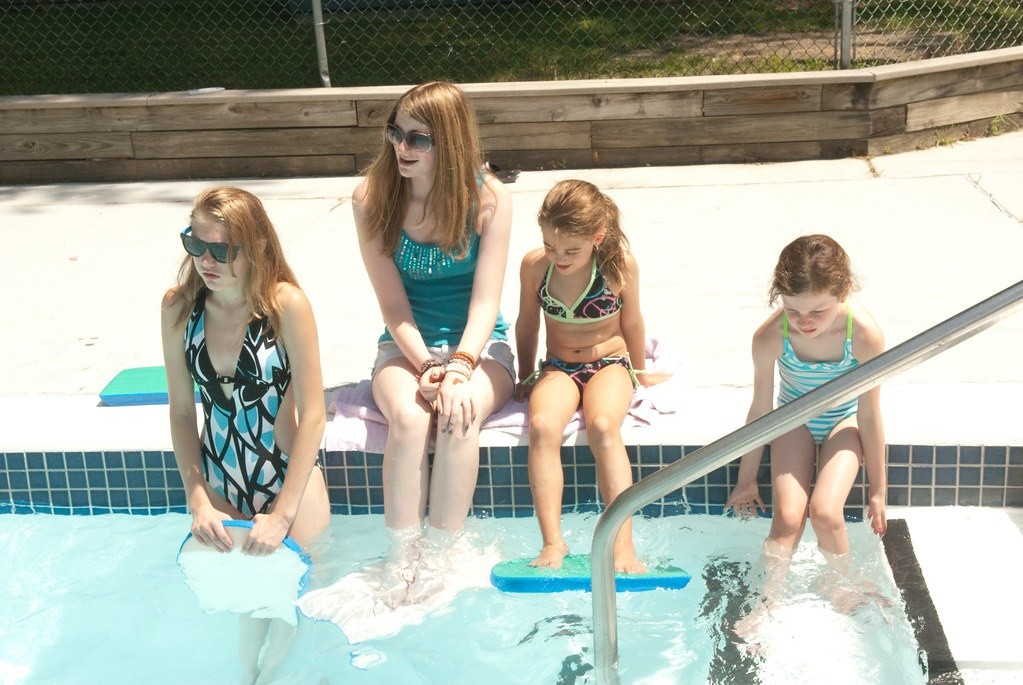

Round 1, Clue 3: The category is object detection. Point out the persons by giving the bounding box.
[725,234,886,555]
[161,187,332,557]
[512,180,670,572]
[352,82,516,530]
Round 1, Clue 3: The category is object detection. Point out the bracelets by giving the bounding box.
[446,351,474,381]
[420,358,442,374]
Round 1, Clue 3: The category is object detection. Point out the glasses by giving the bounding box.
[384,123,435,153]
[180,226,244,263]
